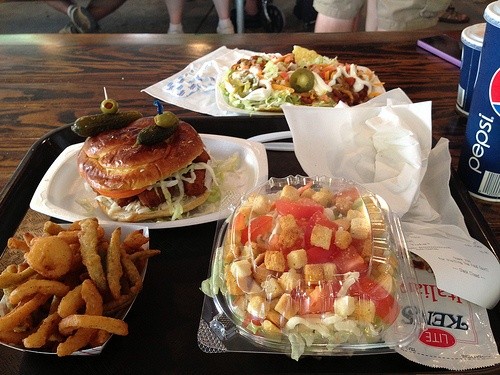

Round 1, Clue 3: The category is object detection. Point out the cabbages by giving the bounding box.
[200,245,388,361]
[220,44,372,110]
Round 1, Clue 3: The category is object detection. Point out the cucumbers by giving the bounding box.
[71,111,144,136]
[137,124,178,148]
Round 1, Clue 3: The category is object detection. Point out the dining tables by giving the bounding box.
[0,29,500,375]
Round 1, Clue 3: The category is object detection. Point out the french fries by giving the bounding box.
[1,217,160,355]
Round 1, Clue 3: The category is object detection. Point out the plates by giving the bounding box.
[29,134,270,230]
[218,59,385,116]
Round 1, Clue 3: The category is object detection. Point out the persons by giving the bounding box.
[312,0,452,33]
[230,0,318,29]
[45,0,127,34]
[165,0,236,35]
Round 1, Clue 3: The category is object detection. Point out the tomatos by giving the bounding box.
[240,196,394,317]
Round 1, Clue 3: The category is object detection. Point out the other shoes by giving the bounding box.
[293,4,317,22]
[58,24,79,35]
[229,7,263,29]
[441,6,471,23]
[66,5,101,35]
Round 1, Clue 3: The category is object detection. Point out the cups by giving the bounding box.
[455,22,487,117]
[457,1,500,203]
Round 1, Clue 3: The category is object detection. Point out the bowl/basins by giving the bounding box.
[198,175,426,359]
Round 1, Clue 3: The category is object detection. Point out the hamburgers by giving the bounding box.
[77,116,240,222]
